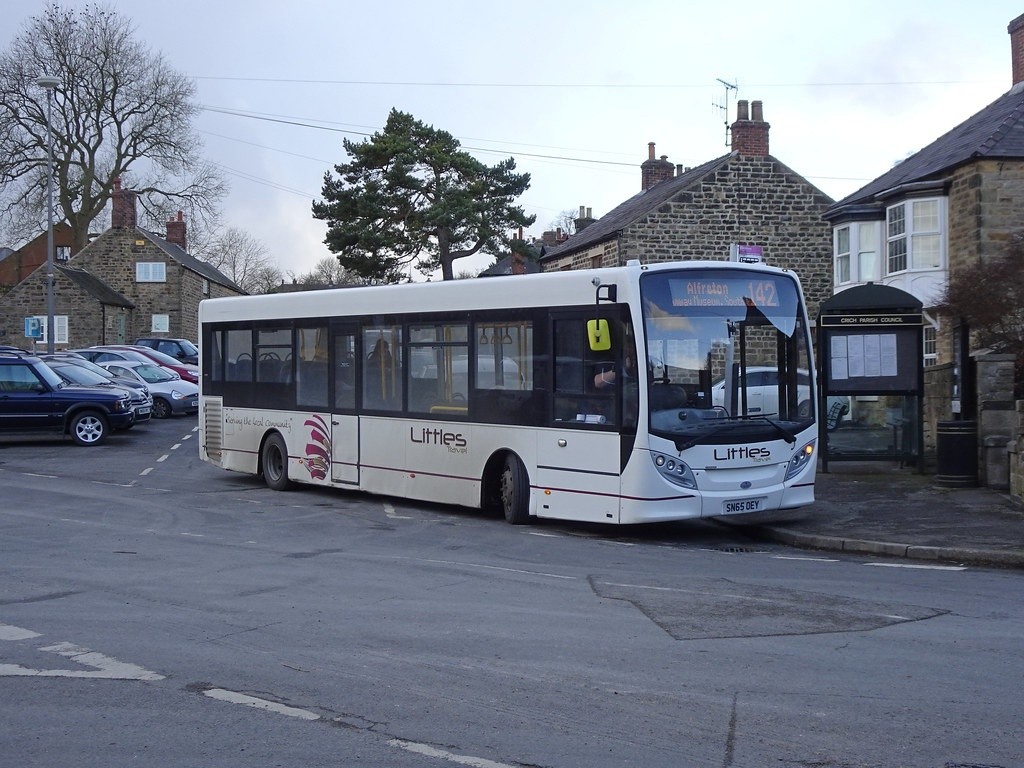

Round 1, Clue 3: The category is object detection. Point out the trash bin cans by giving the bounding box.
[937,420,979,487]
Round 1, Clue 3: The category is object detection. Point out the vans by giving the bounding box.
[444,352,526,405]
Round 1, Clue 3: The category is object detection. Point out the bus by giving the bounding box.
[197,257,819,528]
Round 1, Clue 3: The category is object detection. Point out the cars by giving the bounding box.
[416,365,439,379]
[396,351,436,375]
[349,329,395,354]
[0,337,238,447]
[364,343,416,367]
[711,363,852,423]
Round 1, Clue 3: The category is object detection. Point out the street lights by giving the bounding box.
[32,74,62,354]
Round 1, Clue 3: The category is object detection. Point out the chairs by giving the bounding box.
[231,352,685,427]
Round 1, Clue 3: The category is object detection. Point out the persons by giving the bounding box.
[370,338,394,371]
[593,333,654,394]
